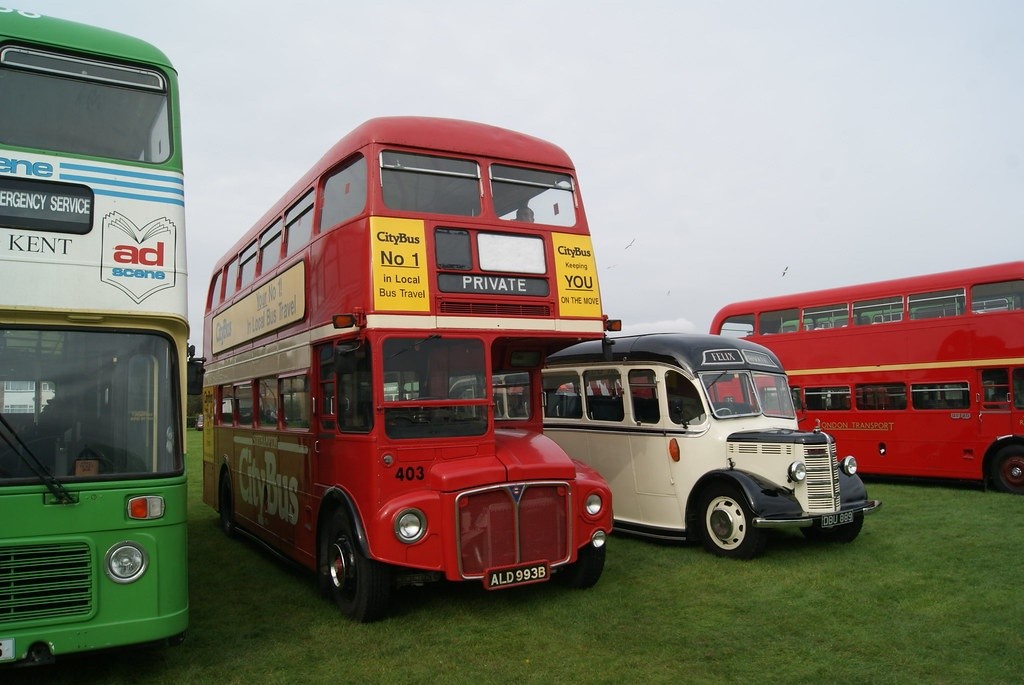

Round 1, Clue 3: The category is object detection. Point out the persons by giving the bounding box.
[516,208,534,223]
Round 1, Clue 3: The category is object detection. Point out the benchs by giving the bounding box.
[523,395,568,416]
[555,395,613,418]
[496,395,526,418]
[588,398,659,423]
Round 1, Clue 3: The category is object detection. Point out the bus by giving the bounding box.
[709,260,1024,494]
[204,117,622,619]
[0,5,205,669]
[444,333,882,554]
[493,373,657,398]
